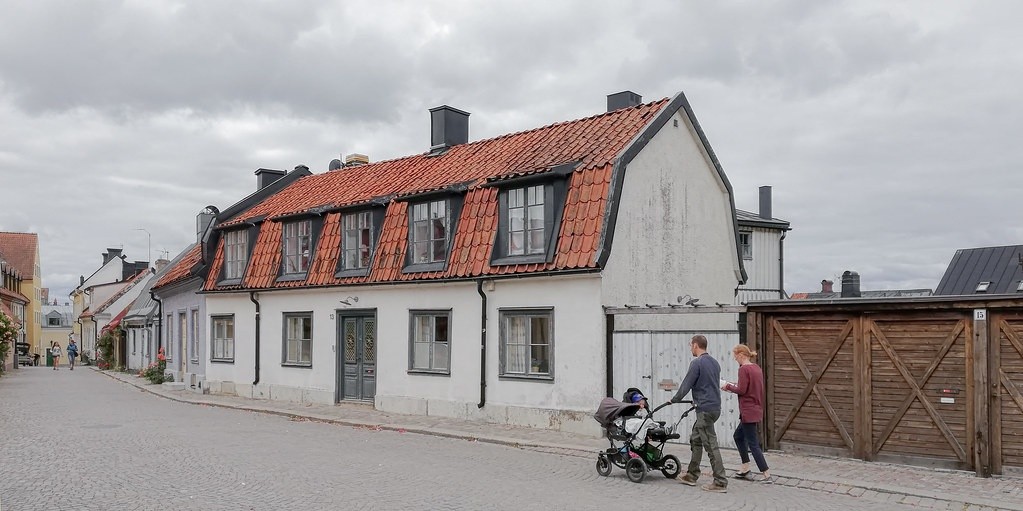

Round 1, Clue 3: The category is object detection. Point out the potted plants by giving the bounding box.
[532,358,546,372]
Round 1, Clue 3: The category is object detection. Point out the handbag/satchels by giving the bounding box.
[74,351,78,357]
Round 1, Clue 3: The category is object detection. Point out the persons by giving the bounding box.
[668,336,728,494]
[67,340,78,371]
[33,354,40,366]
[50,342,64,370]
[721,343,775,484]
[632,393,648,419]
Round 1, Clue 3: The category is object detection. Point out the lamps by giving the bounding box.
[677,295,700,306]
[339,296,358,306]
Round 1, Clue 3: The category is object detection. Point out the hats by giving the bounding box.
[632,393,648,403]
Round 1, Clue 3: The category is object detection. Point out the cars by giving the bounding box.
[17,342,33,366]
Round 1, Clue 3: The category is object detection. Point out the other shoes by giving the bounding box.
[753,476,773,484]
[732,470,751,479]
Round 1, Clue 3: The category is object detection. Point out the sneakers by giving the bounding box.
[701,482,727,493]
[677,473,696,486]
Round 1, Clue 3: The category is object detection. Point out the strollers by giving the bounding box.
[596,388,696,484]
